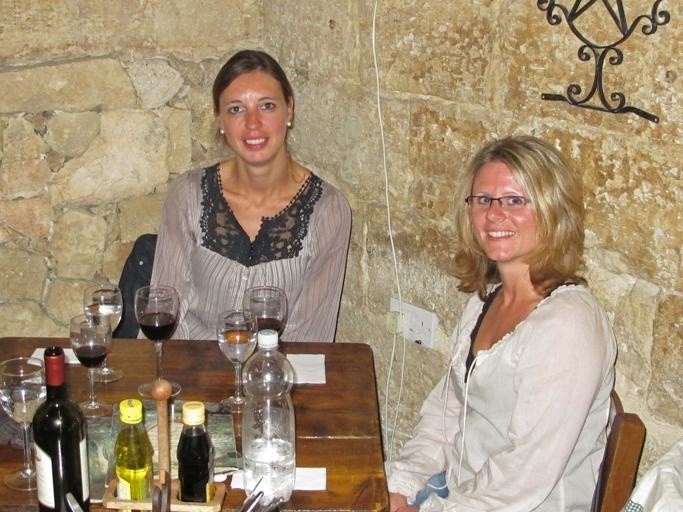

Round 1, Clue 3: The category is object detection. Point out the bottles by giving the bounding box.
[241,328,294,508]
[33,345,213,512]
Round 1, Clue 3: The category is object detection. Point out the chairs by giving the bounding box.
[596,391,647,509]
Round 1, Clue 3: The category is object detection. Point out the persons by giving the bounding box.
[385,136,616,512]
[138,50,352,343]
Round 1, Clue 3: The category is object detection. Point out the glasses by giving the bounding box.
[465,196,532,211]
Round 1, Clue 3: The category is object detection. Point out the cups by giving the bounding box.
[241,286,288,339]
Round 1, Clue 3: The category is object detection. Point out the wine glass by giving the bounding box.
[69,284,180,418]
[0,357,33,493]
[216,309,258,414]
